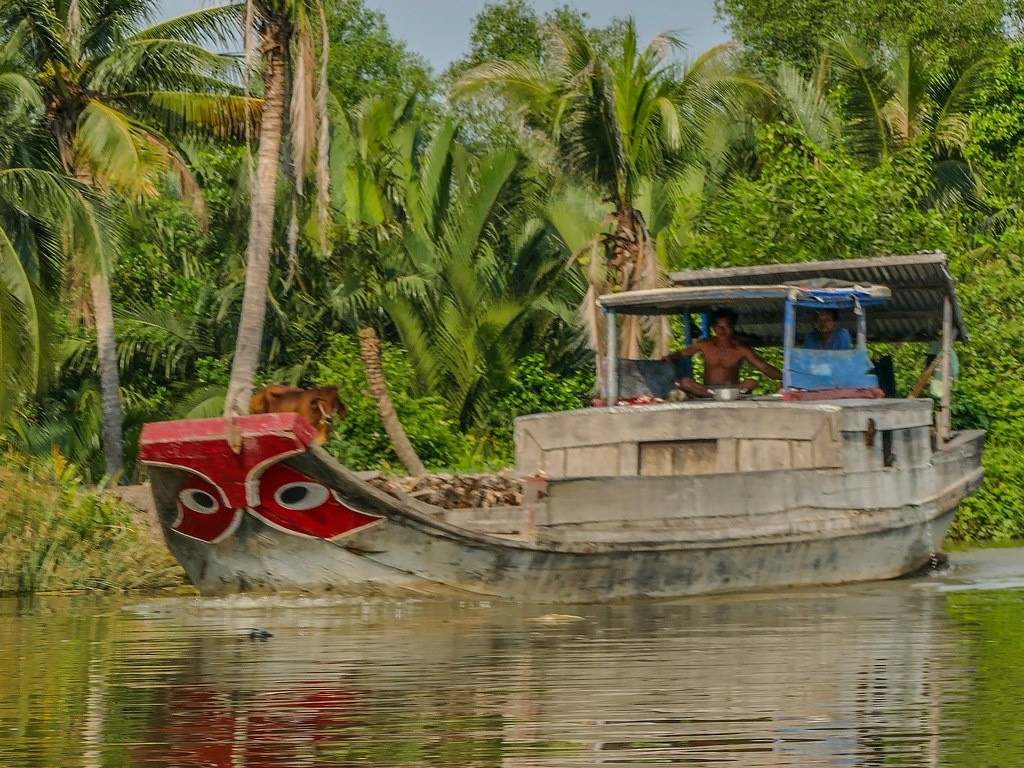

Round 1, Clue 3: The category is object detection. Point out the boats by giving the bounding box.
[138,251,985,603]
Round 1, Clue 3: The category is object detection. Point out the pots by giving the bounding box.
[706,386,739,403]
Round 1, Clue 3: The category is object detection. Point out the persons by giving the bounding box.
[803,308,854,350]
[662,308,783,398]
